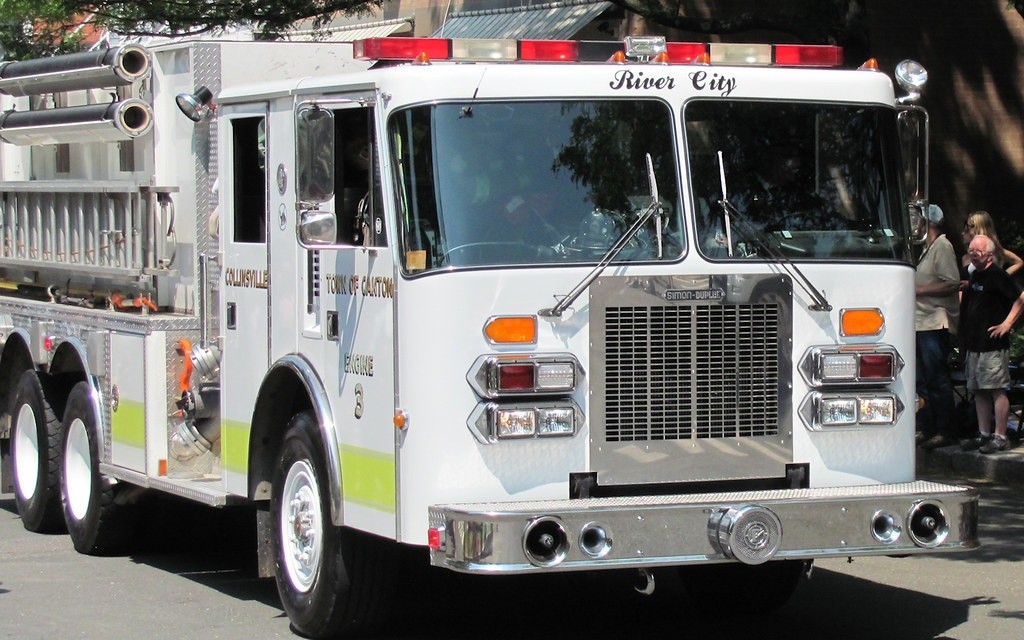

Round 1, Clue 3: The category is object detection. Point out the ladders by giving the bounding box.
[0,181,180,282]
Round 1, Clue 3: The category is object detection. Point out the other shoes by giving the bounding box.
[922,434,943,448]
[964,432,995,450]
[978,433,1012,452]
[915,431,924,443]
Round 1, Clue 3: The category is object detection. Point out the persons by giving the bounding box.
[915,204,1024,454]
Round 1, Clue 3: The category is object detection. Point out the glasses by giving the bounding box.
[967,248,993,258]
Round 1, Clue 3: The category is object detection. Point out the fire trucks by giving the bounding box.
[0,32,983,640]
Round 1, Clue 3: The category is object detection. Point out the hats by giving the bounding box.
[920,203,944,222]
